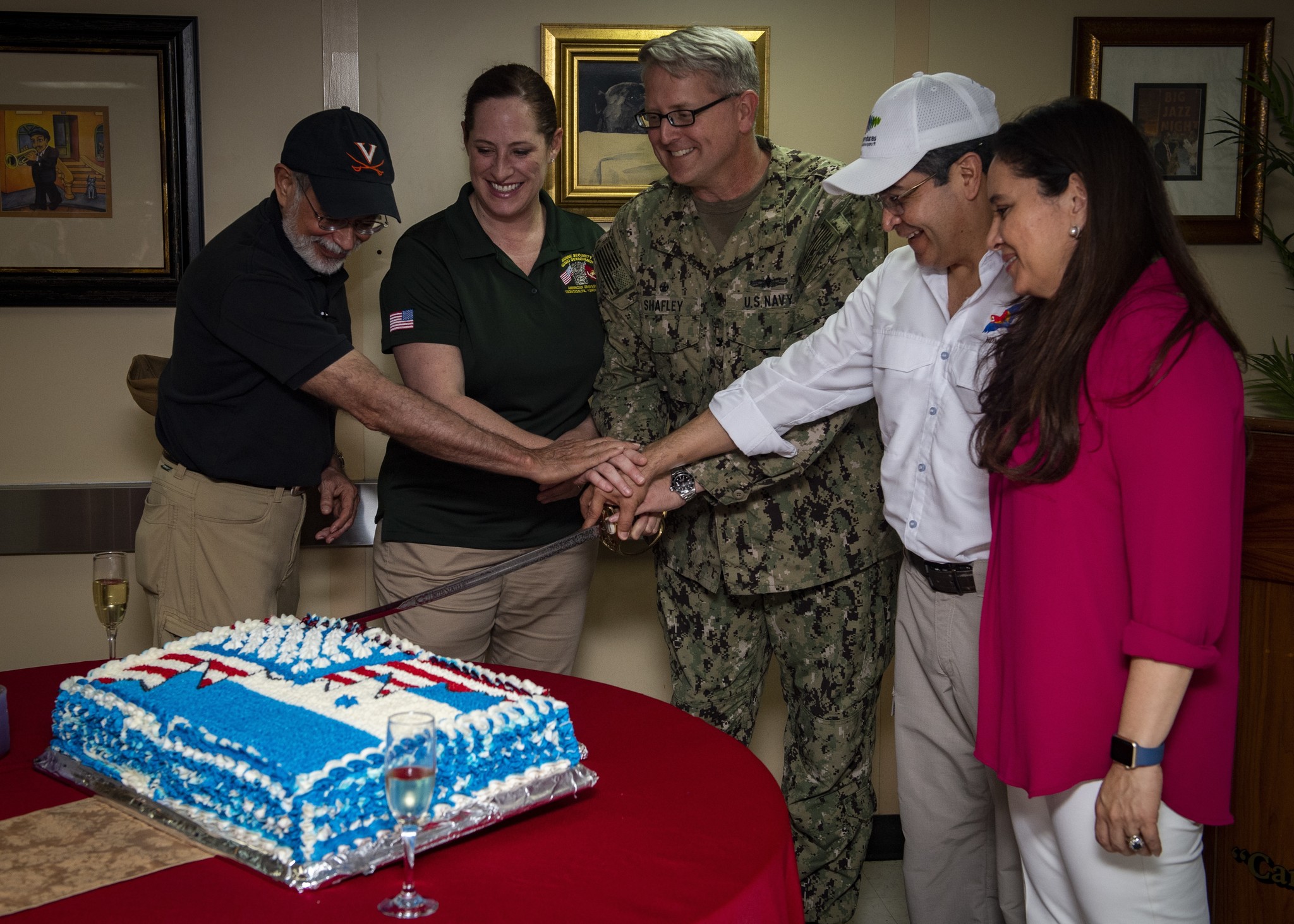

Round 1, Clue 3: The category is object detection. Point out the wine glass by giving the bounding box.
[91,552,130,661]
[380,713,437,919]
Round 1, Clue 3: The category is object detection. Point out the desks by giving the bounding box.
[1,656,807,924]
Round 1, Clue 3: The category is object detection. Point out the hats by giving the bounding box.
[820,71,1001,196]
[279,106,402,224]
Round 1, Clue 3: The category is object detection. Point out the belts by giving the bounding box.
[906,549,976,596]
[161,446,318,497]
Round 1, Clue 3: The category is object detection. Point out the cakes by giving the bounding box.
[48,606,583,867]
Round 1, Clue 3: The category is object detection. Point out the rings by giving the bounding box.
[1125,834,1144,852]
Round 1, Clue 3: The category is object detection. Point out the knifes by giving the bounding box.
[337,506,665,626]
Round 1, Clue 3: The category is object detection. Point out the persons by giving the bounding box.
[977,94,1248,924]
[1154,131,1175,176]
[133,104,641,649]
[370,57,648,678]
[586,25,904,924]
[578,69,1026,923]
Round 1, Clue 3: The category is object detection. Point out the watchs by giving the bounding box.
[669,466,697,502]
[1108,730,1165,771]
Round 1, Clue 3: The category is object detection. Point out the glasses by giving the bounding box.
[870,141,986,216]
[293,172,389,235]
[634,91,742,129]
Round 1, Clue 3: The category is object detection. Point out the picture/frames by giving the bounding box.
[540,22,769,224]
[0,10,206,307]
[1070,15,1275,247]
